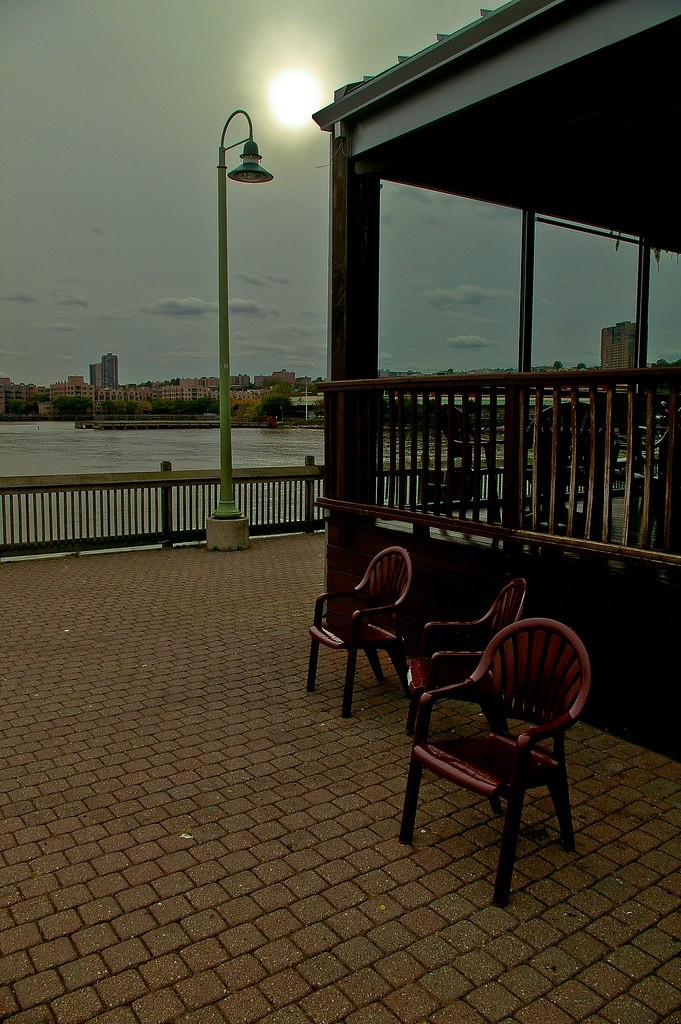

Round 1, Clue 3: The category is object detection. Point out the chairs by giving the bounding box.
[399,618,591,909]
[405,577,527,737]
[442,397,681,540]
[306,546,414,720]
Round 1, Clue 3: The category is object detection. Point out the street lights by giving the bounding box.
[206,109,273,551]
